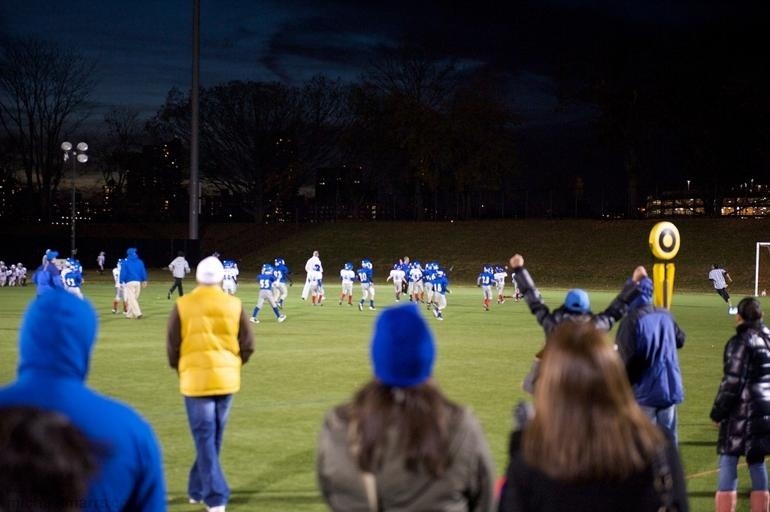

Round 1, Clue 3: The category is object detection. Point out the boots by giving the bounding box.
[750,490,770,511]
[715,490,737,512]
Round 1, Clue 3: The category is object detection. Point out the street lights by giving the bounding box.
[59,138,89,256]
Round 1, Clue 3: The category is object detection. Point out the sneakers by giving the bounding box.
[249,317,260,324]
[358,302,375,312]
[206,504,226,512]
[312,303,322,306]
[483,303,489,311]
[338,302,353,306]
[277,302,282,309]
[278,314,286,323]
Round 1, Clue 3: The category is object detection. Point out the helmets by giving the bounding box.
[484,265,503,273]
[394,261,446,277]
[0,261,23,271]
[224,259,233,266]
[344,262,353,269]
[711,263,719,269]
[625,276,653,296]
[264,257,282,272]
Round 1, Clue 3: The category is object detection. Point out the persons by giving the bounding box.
[250,258,293,324]
[301,250,325,306]
[112,249,147,319]
[708,263,733,307]
[168,254,190,299]
[6,408,93,503]
[0,289,167,512]
[509,252,649,395]
[338,258,376,310]
[478,265,524,311]
[96,251,106,274]
[494,314,691,511]
[167,259,255,511]
[618,277,686,464]
[1,261,26,287]
[33,249,84,294]
[387,257,452,320]
[314,301,494,512]
[222,257,239,295]
[706,297,770,511]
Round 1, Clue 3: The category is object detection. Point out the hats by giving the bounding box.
[47,251,60,260]
[565,288,590,312]
[373,304,435,384]
[361,259,368,263]
[196,255,224,284]
[127,248,136,255]
